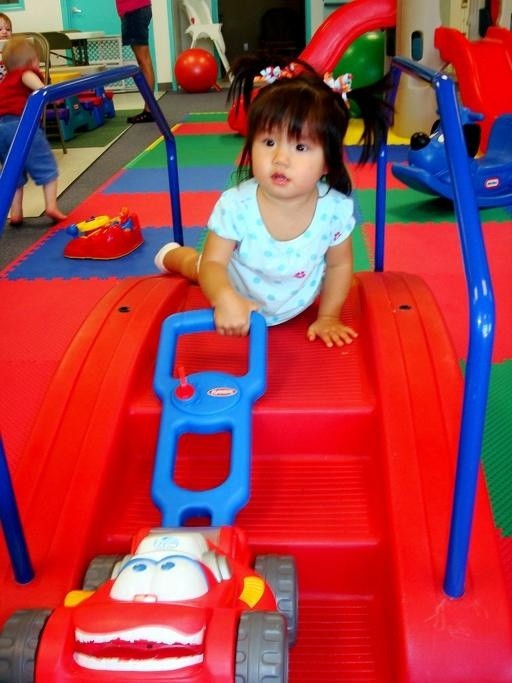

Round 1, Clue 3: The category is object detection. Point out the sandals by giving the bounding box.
[127,110,156,123]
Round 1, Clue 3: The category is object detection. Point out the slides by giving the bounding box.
[228,0,396,137]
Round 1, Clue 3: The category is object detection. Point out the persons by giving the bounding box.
[154,45,396,348]
[116,0,155,123]
[0,13,67,225]
